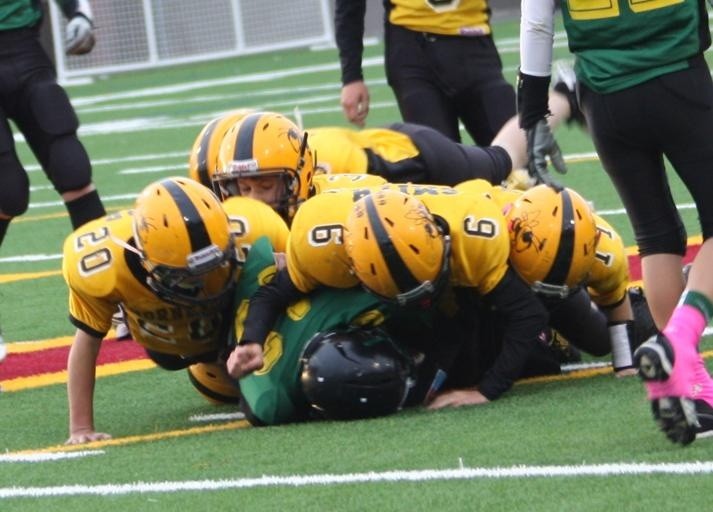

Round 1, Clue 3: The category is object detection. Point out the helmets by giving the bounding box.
[295,323,416,424]
[185,109,316,222]
[126,172,238,308]
[501,180,598,302]
[345,191,455,309]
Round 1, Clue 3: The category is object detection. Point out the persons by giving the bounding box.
[511,0,713,445]
[0,0,128,341]
[189,63,586,231]
[331,0,538,181]
[62,178,661,446]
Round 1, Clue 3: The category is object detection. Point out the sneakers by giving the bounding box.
[112,304,139,345]
[558,60,588,133]
[630,332,712,446]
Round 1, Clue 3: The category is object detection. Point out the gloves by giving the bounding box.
[62,14,96,58]
[521,119,567,193]
